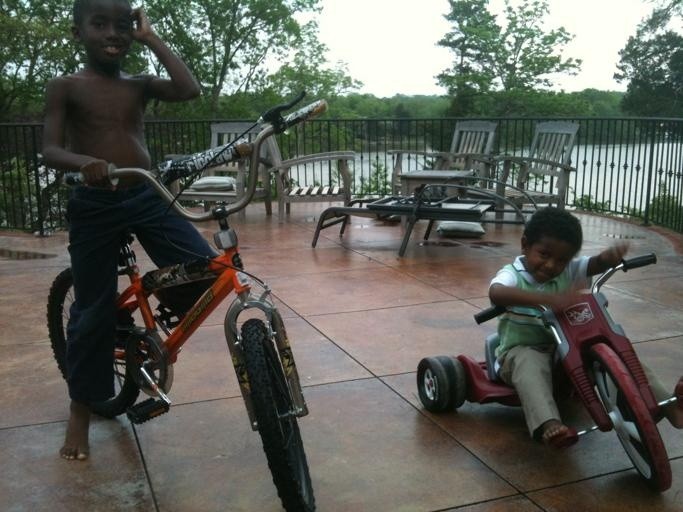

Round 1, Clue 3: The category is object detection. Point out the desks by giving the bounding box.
[313,176,539,257]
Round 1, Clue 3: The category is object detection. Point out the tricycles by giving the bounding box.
[417,252,679,494]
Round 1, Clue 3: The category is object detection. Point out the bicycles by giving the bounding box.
[47,84,325,512]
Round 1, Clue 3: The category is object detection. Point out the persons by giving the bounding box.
[39,1,226,462]
[488,205,631,447]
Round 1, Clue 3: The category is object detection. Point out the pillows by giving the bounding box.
[437,221,485,237]
[192,176,235,191]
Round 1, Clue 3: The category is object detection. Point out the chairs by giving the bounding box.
[164,123,272,224]
[388,122,498,195]
[455,122,580,229]
[264,123,354,224]
